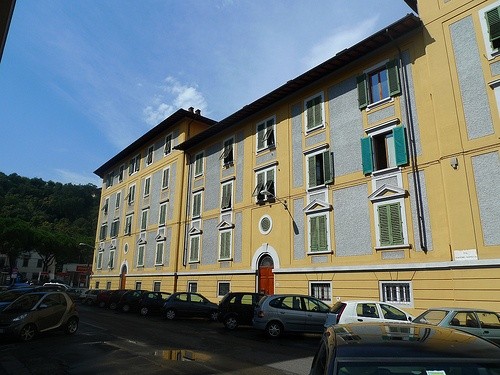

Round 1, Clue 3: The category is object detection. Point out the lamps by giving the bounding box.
[260,189,288,209]
[78,242,96,249]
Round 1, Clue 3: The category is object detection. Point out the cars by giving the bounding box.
[0,283,173,315]
[216,292,292,329]
[323,300,427,329]
[252,294,331,339]
[160,292,219,322]
[0,292,80,342]
[308,322,499,375]
[409,308,500,341]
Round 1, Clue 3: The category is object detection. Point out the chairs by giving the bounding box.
[296,298,301,310]
[363,305,378,318]
[452,318,461,326]
